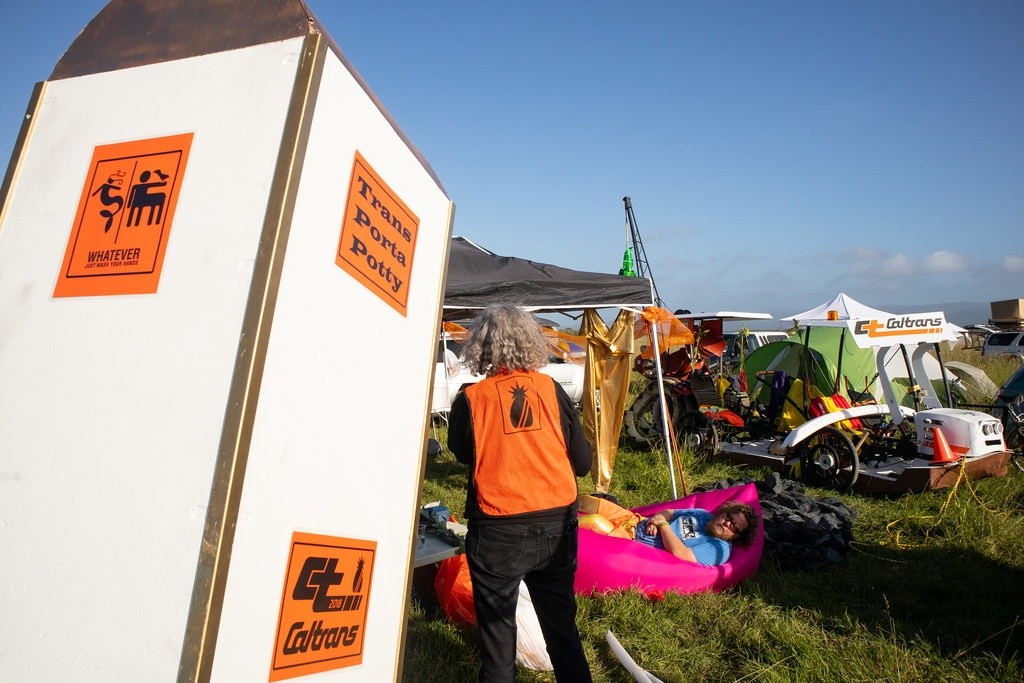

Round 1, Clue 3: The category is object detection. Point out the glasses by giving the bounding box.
[723,511,742,535]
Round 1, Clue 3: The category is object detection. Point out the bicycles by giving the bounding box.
[947,376,1024,476]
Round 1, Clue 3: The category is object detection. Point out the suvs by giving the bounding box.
[432,329,602,426]
[980,331,1024,368]
[710,329,792,374]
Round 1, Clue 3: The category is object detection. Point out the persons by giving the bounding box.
[446,298,595,683]
[578,494,758,565]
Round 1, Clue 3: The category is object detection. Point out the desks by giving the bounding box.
[413,520,469,568]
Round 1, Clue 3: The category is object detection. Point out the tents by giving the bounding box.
[443,233,677,501]
[779,293,968,340]
[730,325,972,440]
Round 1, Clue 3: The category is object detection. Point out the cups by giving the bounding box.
[416,525,426,549]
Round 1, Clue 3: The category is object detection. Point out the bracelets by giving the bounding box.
[657,522,669,527]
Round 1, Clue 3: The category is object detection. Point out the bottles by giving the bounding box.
[755,398,766,413]
[623,246,634,276]
